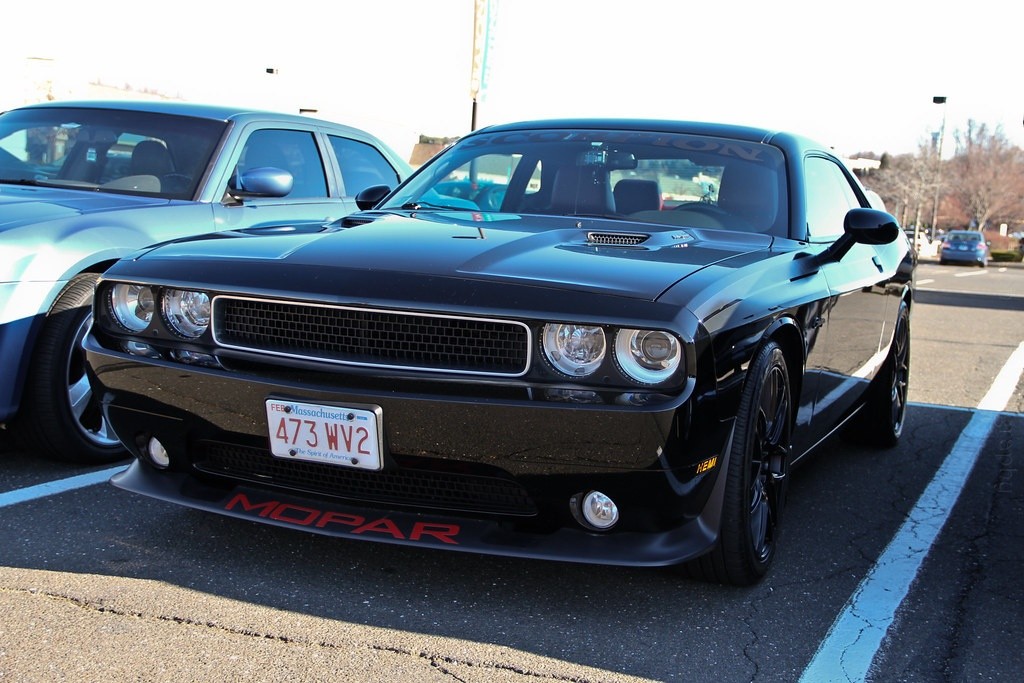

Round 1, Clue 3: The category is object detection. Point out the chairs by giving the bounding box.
[614,178,663,215]
[128,140,191,194]
[717,160,778,220]
[545,162,617,215]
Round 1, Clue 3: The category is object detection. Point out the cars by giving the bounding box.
[904,229,930,259]
[938,230,992,267]
[83,120,919,585]
[0,102,478,467]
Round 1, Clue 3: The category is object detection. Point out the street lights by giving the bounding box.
[930,94,948,261]
